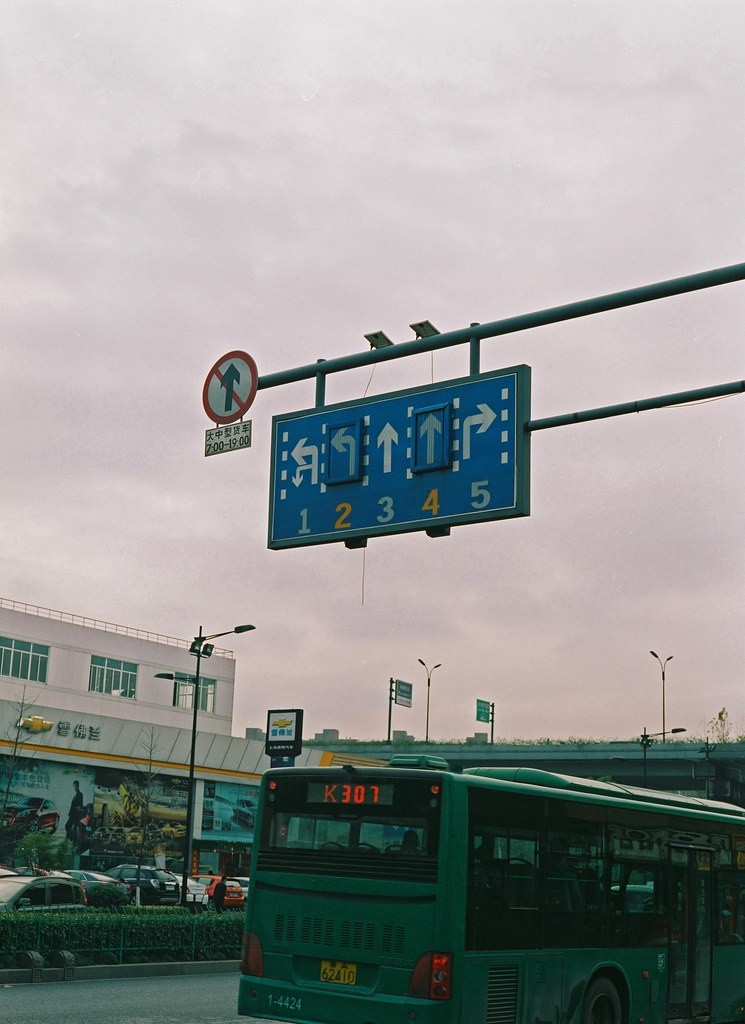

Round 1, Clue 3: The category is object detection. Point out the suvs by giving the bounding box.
[103,862,181,907]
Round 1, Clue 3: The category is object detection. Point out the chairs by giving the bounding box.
[322,841,601,916]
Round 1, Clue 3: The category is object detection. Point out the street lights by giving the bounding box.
[417,658,442,743]
[649,650,675,744]
[154,622,258,908]
[607,726,689,788]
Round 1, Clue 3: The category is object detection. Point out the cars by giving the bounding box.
[190,874,245,909]
[0,863,74,878]
[0,795,60,834]
[86,821,186,847]
[62,869,128,904]
[227,876,250,902]
[175,872,209,911]
[231,792,258,828]
[0,874,88,914]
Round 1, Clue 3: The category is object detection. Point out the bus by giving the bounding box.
[236,751,745,1024]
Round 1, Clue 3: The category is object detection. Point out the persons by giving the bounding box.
[208,868,228,914]
[116,878,128,896]
[393,829,424,859]
[65,782,84,839]
